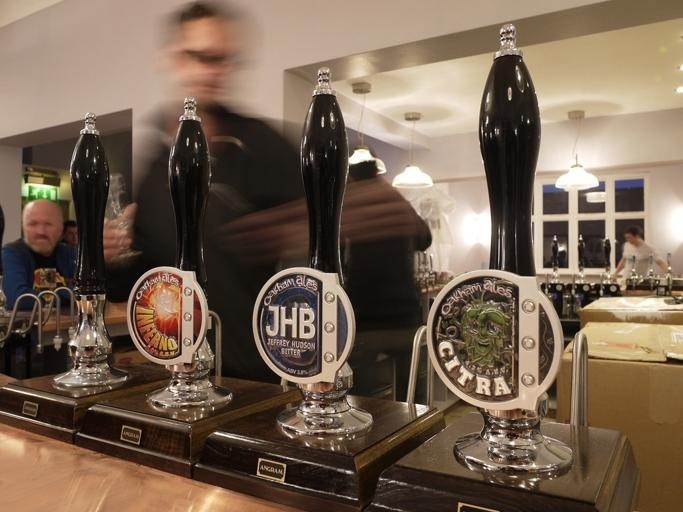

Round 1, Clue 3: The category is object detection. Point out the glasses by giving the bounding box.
[181,49,234,66]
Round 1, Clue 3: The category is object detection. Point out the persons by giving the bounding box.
[0,198,80,312]
[103,2,433,394]
[60,219,80,247]
[607,224,677,283]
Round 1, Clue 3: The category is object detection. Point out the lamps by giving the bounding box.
[391,111,432,190]
[555,111,599,191]
[347,82,387,175]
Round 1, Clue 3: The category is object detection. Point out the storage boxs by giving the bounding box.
[555,322,683,512]
[578,297,683,332]
